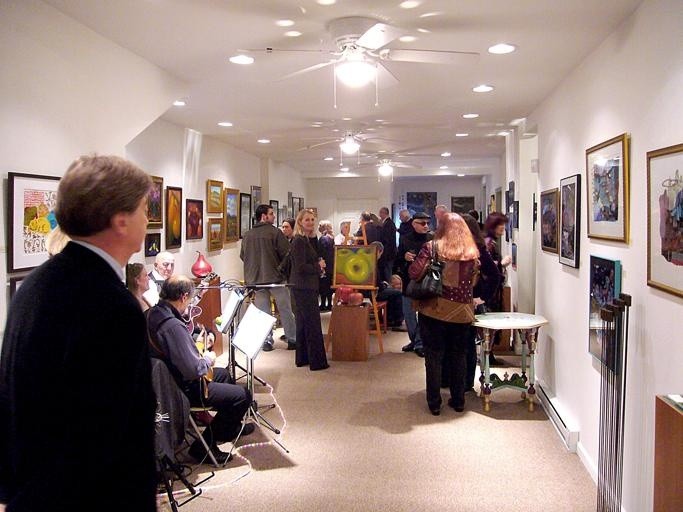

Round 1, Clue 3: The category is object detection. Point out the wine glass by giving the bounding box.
[316,257,325,278]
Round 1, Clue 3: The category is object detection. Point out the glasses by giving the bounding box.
[414,221,431,226]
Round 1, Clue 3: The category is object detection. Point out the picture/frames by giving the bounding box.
[6,275,27,296]
[333,244,377,285]
[582,133,633,245]
[645,142,682,297]
[142,174,309,260]
[483,179,519,241]
[6,170,67,272]
[536,188,560,257]
[554,173,582,268]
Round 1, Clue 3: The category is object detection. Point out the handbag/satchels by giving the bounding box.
[406,240,442,302]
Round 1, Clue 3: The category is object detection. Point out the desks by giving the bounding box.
[471,311,546,414]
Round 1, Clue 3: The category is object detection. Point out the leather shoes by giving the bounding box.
[287,341,297,350]
[430,408,441,416]
[402,342,415,352]
[188,446,233,465]
[238,422,255,436]
[447,398,465,413]
[262,342,273,351]
[414,347,426,358]
[391,325,408,332]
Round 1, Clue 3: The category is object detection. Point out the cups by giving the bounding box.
[409,249,414,261]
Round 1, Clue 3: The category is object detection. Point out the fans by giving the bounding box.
[292,115,398,161]
[353,149,420,181]
[237,17,478,88]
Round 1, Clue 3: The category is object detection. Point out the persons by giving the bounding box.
[240,204,353,371]
[0,155,157,511]
[355,205,512,416]
[126,251,254,462]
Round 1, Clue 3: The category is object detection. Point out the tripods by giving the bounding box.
[248,358,280,435]
[224,321,266,386]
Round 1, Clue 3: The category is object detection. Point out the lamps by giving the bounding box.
[335,139,361,158]
[333,59,375,92]
[374,164,396,178]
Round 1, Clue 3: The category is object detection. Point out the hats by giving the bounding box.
[412,212,432,220]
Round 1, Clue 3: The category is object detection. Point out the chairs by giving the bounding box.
[369,300,388,333]
[149,360,221,468]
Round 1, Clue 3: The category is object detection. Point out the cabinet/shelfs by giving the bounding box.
[187,277,223,356]
